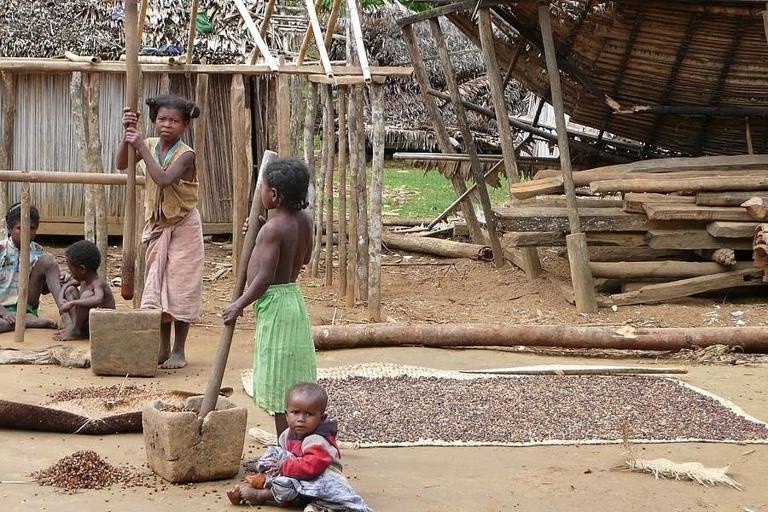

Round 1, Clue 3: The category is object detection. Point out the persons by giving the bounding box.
[0,202,71,334]
[53,240,115,341]
[226,383,342,505]
[222,159,315,472]
[115,94,204,369]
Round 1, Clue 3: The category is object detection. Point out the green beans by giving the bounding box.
[50,387,143,403]
[34,451,197,493]
[318,375,768,443]
